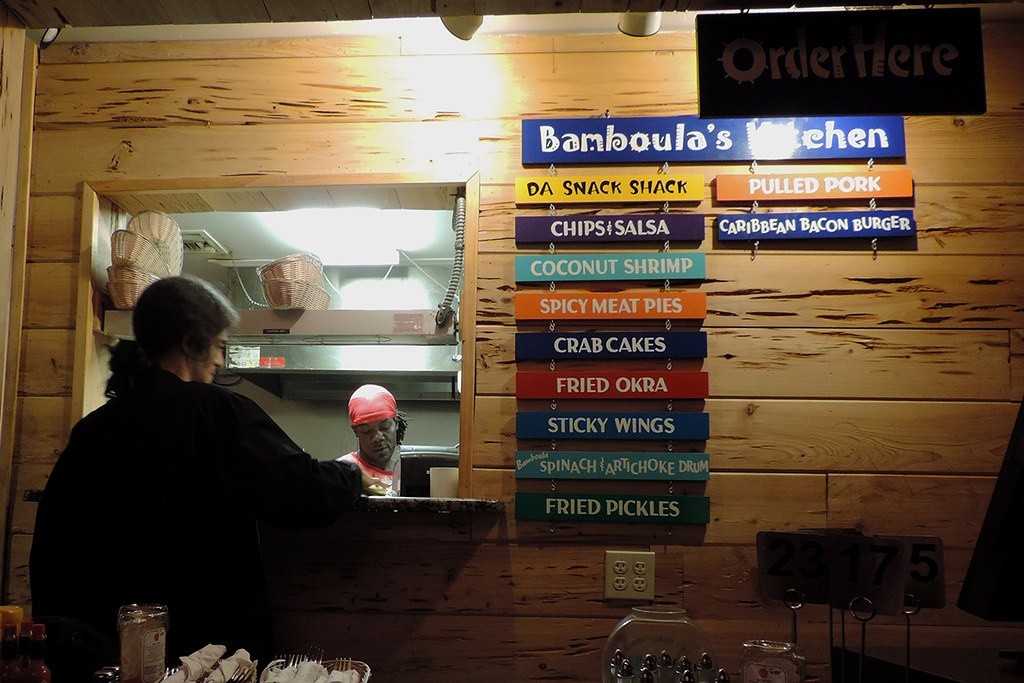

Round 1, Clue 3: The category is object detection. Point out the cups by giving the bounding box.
[429,468,459,498]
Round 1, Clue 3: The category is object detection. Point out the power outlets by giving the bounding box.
[604,550,655,601]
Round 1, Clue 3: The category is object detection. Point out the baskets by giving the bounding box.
[257,252,325,274]
[106,266,161,284]
[260,659,370,683]
[110,229,172,280]
[258,259,325,288]
[261,269,331,311]
[106,282,150,310]
[126,209,183,273]
[151,659,257,683]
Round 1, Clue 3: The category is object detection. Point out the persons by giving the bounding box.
[29,276,392,675]
[332,384,411,497]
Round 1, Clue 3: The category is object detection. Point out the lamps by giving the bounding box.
[439,15,484,42]
[617,11,662,37]
[25,28,61,51]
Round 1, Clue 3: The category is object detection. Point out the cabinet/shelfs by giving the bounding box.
[829,644,1024,683]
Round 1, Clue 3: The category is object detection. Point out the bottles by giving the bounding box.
[0,604,168,683]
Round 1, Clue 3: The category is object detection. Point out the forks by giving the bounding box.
[163,667,181,680]
[272,646,352,671]
[226,667,254,683]
